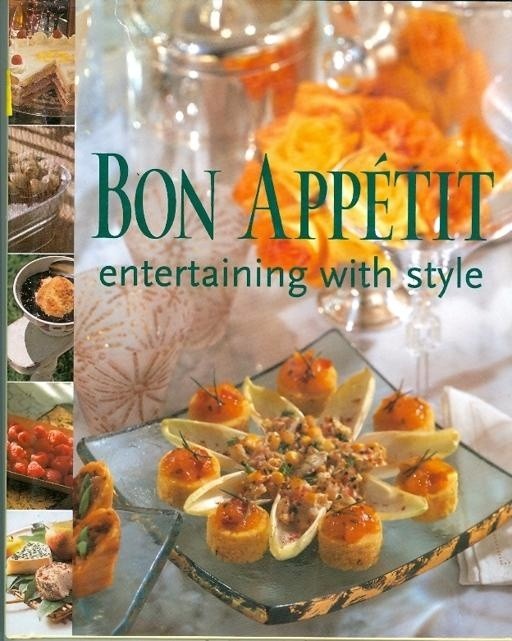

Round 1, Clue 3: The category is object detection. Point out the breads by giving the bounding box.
[36,276,74,317]
[12,62,75,115]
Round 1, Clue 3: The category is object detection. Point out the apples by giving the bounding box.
[45,521,76,560]
[6,537,24,558]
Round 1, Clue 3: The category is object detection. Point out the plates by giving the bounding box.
[78,327,511,636]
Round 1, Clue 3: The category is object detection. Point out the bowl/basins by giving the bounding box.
[12,255,76,338]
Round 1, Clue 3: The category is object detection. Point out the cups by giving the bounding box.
[73,184,254,437]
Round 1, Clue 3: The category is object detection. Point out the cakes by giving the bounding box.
[73,508,120,597]
[190,384,250,434]
[373,392,434,434]
[206,497,268,564]
[318,505,382,570]
[8,541,52,574]
[396,455,457,521]
[157,448,221,506]
[36,562,73,600]
[276,354,337,418]
[73,462,112,523]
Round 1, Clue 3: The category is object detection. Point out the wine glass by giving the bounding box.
[326,134,512,409]
